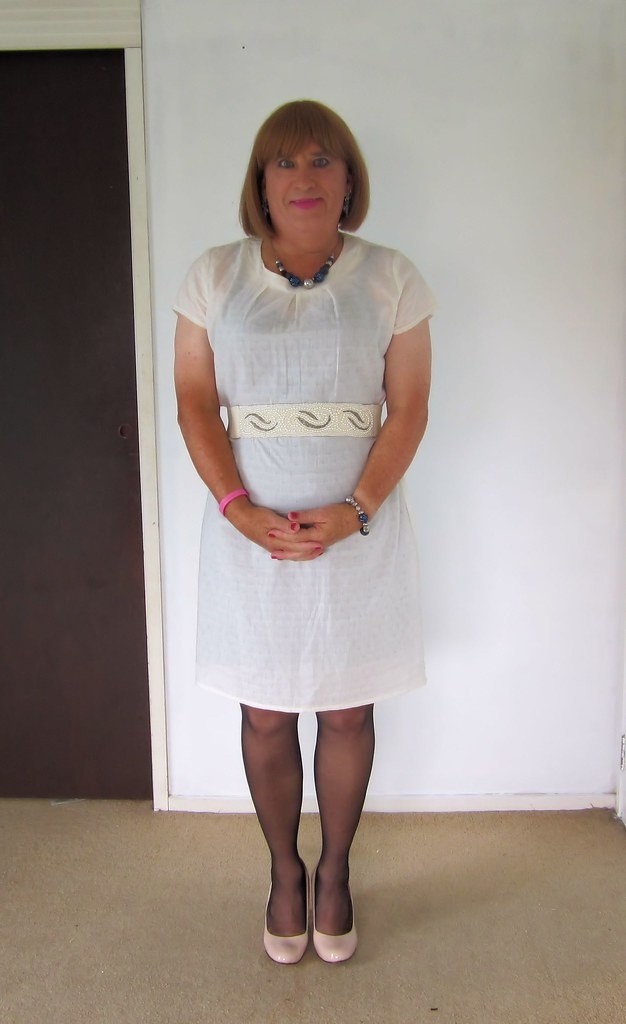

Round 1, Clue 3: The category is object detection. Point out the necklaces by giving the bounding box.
[270,238,340,288]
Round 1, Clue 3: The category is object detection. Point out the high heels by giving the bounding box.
[263,858,309,965]
[311,865,358,963]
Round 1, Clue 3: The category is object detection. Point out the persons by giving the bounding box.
[172,101,432,964]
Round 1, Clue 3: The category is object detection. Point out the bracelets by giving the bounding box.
[346,496,370,536]
[218,489,249,518]
